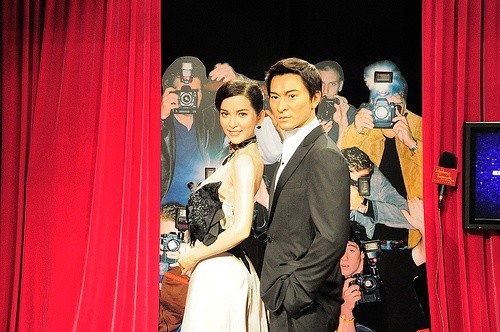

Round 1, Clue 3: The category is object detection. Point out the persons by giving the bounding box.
[260,58,351,332]
[158,56,430,332]
[177,79,270,332]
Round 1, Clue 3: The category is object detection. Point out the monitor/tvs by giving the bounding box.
[463,123,500,231]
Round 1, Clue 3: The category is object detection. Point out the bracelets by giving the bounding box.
[409,143,417,152]
[340,317,354,323]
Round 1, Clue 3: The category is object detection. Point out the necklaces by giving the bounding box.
[222,135,257,165]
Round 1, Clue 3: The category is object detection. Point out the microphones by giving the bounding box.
[433,151,458,208]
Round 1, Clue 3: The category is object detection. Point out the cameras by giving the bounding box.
[159,206,188,252]
[366,98,402,129]
[350,273,382,306]
[317,96,339,122]
[170,85,199,114]
